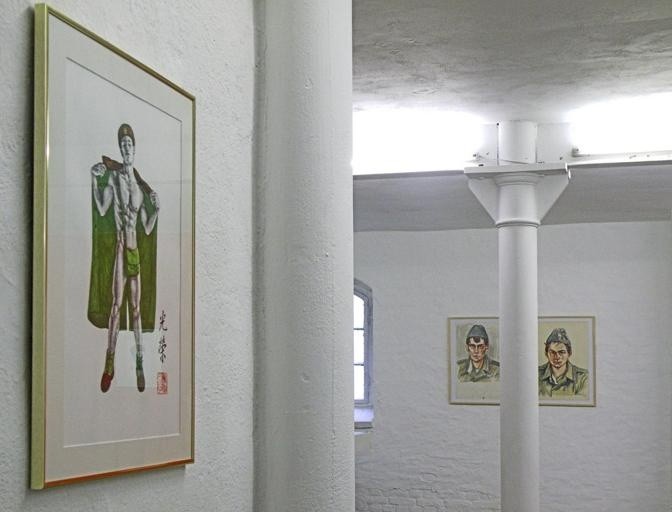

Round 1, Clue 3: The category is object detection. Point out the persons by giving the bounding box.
[539,326,589,401]
[88,121,160,394]
[457,324,500,383]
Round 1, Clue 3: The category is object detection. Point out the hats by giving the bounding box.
[546,327,571,347]
[467,324,489,347]
[118,124,134,149]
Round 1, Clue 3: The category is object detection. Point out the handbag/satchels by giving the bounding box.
[123,244,141,276]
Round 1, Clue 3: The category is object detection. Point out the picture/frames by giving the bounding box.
[26,3,199,492]
[447,316,500,408]
[536,315,596,410]
